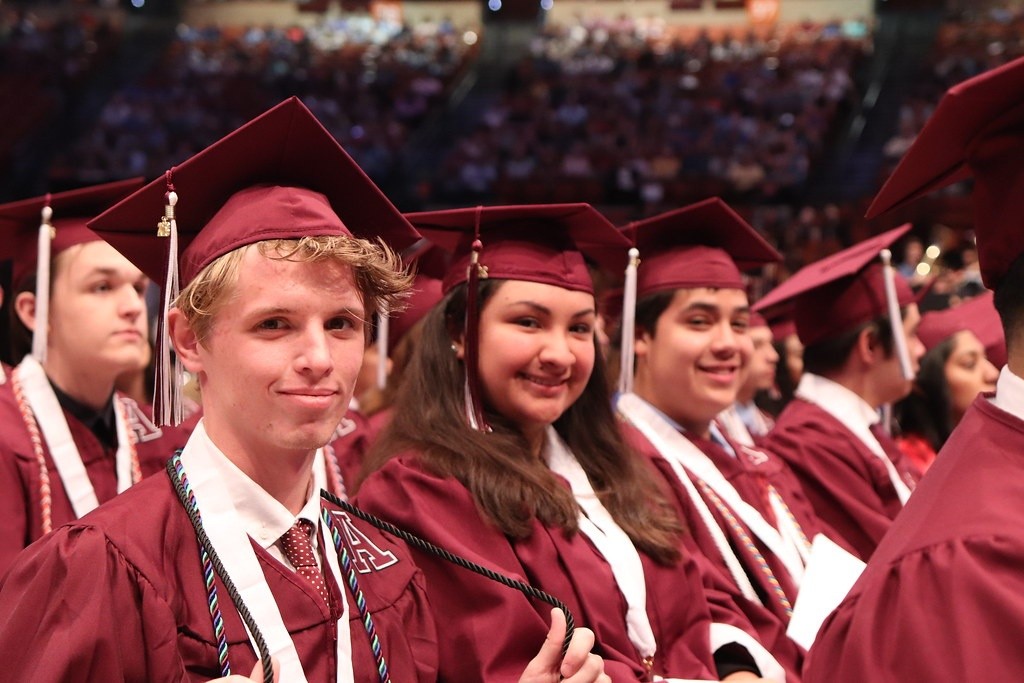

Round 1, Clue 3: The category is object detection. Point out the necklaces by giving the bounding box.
[696,479,810,617]
[172,448,390,683]
[12,364,143,534]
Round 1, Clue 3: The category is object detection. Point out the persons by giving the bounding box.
[0,58,1024,683]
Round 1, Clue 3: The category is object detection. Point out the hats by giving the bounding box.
[376,239,444,390]
[865,55,1024,292]
[398,201,632,434]
[615,195,784,393]
[87,95,424,426]
[747,223,917,382]
[0,177,145,363]
[916,289,1007,370]
